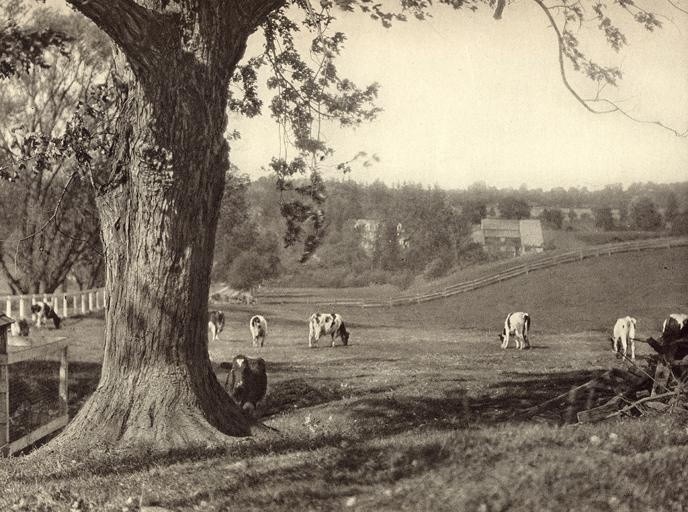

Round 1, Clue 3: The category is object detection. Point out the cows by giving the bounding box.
[219,354,268,408]
[208,310,225,341]
[249,314,269,348]
[661,312,688,335]
[31,301,61,330]
[306,312,350,348]
[498,311,531,350]
[609,315,638,362]
